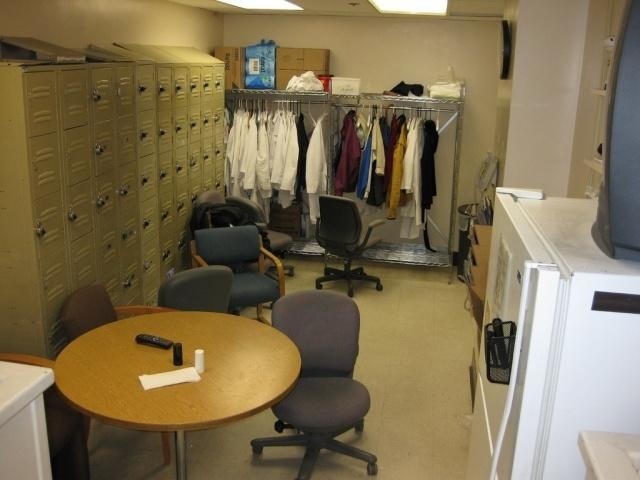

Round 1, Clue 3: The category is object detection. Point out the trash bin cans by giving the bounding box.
[458,203,481,283]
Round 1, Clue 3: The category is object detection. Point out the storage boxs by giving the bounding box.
[214,47,246,90]
[275,46,360,95]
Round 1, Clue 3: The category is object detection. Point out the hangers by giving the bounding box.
[227,98,440,132]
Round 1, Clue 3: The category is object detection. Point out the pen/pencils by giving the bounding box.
[488,318,509,369]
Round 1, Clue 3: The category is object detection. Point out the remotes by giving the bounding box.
[135,334,173,349]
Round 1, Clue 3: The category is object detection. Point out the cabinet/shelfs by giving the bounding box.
[583,34,617,197]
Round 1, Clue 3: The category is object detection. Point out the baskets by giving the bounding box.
[486,321,516,385]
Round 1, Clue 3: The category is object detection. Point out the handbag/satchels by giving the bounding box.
[190,203,271,253]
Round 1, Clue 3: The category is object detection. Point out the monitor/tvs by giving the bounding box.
[591,1,640,264]
